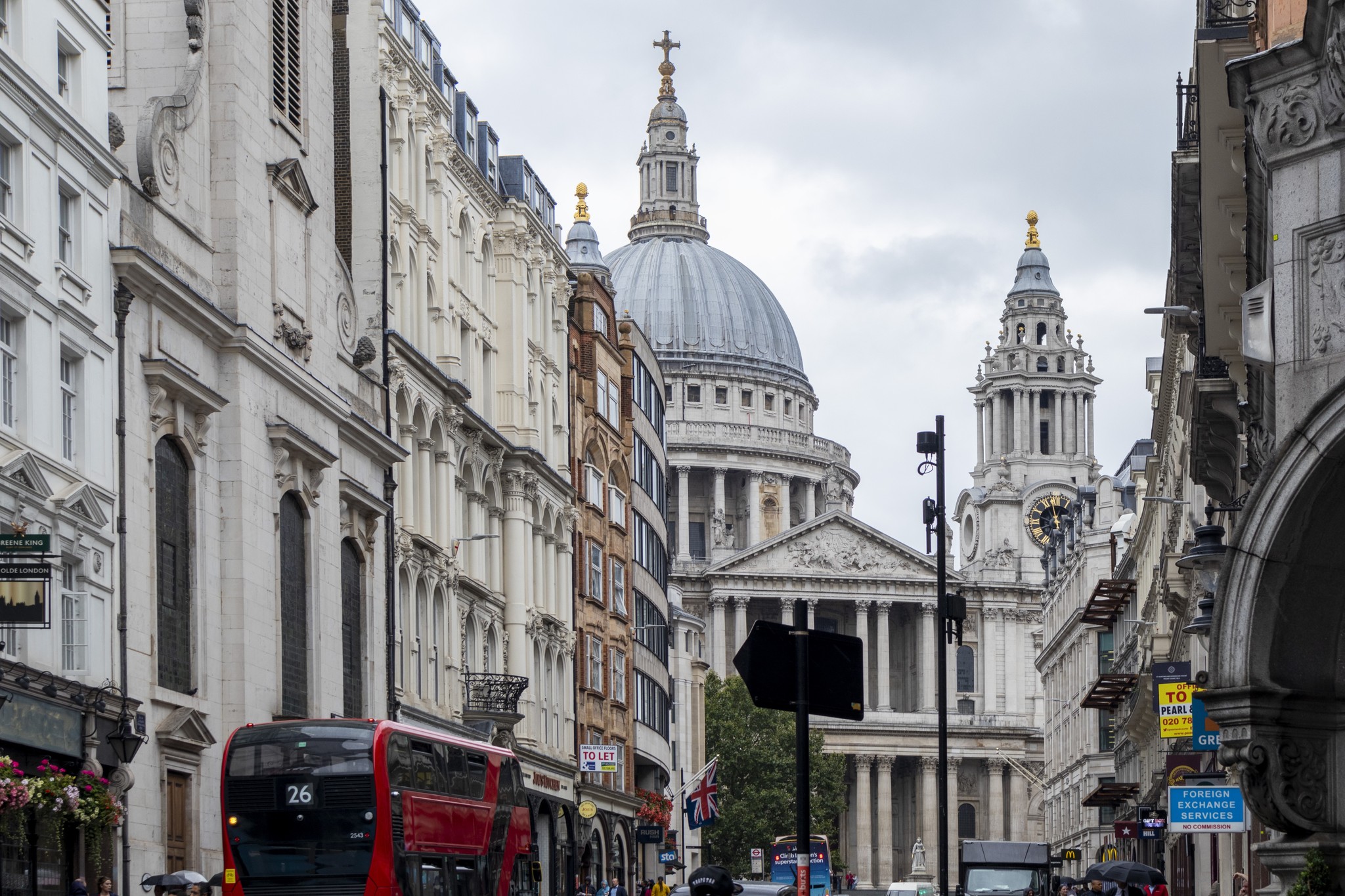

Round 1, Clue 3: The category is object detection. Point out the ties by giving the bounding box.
[1121,890,1125,896]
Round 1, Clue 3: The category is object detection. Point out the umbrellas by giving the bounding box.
[1085,863,1114,882]
[139,874,192,896]
[1060,876,1077,887]
[1098,862,1168,885]
[171,870,208,889]
[208,872,223,886]
[1075,877,1092,885]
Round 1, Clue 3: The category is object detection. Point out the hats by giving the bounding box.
[688,864,744,896]
[648,878,654,883]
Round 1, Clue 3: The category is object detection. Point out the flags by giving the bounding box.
[691,760,720,824]
[685,794,714,831]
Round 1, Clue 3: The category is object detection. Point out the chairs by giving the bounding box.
[264,752,475,799]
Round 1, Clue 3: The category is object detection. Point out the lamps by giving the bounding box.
[629,623,666,642]
[1044,697,1071,710]
[450,532,501,560]
[1141,494,1193,510]
[80,685,150,765]
[0,661,107,715]
[1122,618,1159,627]
[1173,488,1252,655]
[1142,304,1200,329]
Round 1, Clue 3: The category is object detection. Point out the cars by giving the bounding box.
[667,879,800,896]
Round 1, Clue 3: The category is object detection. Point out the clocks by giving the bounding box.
[1021,490,1074,554]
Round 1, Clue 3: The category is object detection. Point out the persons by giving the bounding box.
[95,877,119,896]
[608,878,628,896]
[911,837,926,867]
[1023,887,1034,896]
[646,883,655,896]
[851,873,858,889]
[846,871,854,890]
[688,864,744,896]
[576,877,596,896]
[190,881,212,896]
[636,880,648,896]
[154,885,166,896]
[1051,868,1169,896]
[168,889,186,896]
[647,878,655,889]
[72,878,90,896]
[651,876,671,896]
[596,880,610,896]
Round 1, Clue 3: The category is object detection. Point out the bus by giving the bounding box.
[770,833,833,896]
[219,716,542,896]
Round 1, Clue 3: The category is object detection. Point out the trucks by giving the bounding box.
[955,839,1053,896]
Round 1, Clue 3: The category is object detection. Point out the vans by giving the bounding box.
[886,882,935,896]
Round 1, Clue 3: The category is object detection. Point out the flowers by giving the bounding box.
[634,785,683,862]
[0,753,131,883]
